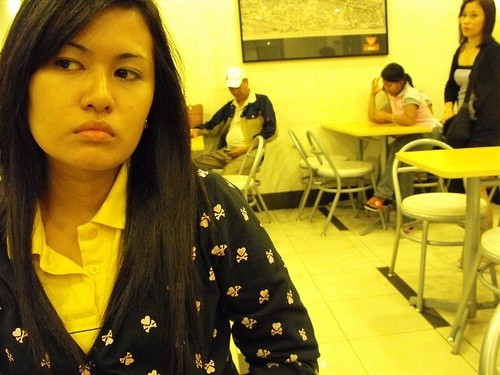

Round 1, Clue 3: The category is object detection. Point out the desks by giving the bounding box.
[395,146,500,355]
[322,113,434,236]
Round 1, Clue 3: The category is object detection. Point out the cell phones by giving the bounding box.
[224,149,231,152]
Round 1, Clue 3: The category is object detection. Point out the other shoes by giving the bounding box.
[402,225,412,234]
[364,197,383,212]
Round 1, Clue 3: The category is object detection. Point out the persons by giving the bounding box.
[363,63,436,238]
[0,0,321,375]
[427,0,500,201]
[188,68,278,174]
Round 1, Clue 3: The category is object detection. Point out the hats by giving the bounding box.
[226,67,247,88]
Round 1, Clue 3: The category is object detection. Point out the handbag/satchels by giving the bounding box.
[443,106,472,148]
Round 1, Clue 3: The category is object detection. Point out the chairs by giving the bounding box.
[448,228,500,343]
[306,130,387,237]
[220,135,275,223]
[287,128,361,222]
[387,138,500,313]
